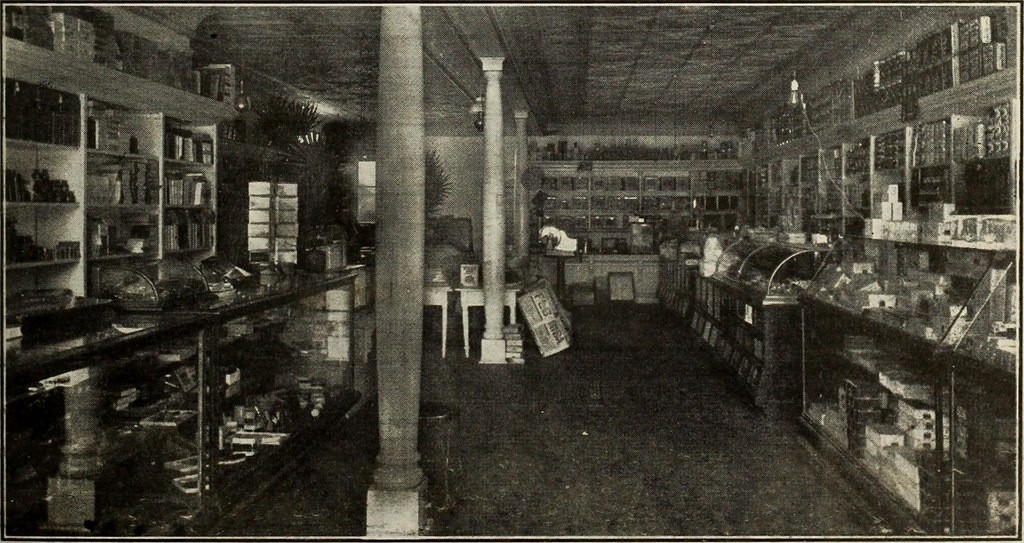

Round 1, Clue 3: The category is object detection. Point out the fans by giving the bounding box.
[539,224,561,250]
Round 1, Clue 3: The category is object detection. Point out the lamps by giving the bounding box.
[470,82,485,114]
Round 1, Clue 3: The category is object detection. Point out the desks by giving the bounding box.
[422,283,454,358]
[454,283,526,359]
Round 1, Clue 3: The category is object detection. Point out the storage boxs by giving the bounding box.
[809,16,1018,537]
[3,4,235,104]
[517,279,574,357]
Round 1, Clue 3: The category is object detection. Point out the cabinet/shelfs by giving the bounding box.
[545,250,575,308]
[3,32,362,536]
[527,66,1017,536]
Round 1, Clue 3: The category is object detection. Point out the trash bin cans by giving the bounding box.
[417,405,454,505]
